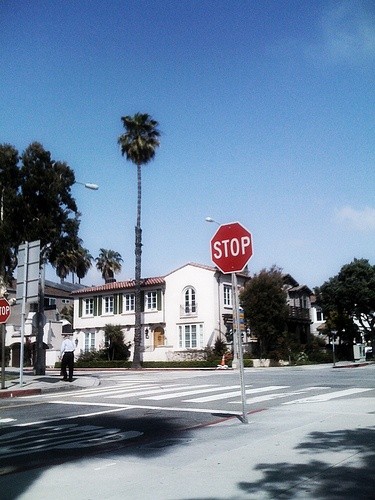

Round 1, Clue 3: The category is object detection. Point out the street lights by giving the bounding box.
[34,180,99,373]
[205,216,222,229]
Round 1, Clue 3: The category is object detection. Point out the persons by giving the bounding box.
[59,334,75,382]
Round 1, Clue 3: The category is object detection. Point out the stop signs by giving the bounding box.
[210,222,253,273]
[0,299,11,324]
